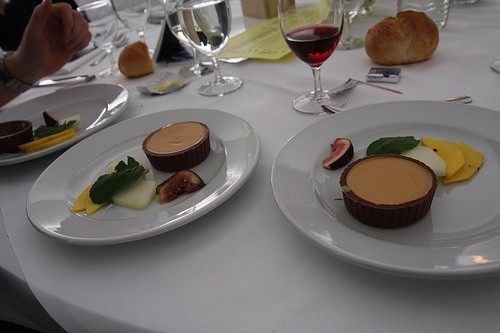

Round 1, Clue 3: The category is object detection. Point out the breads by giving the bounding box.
[364,10,439,65]
[118,40,155,77]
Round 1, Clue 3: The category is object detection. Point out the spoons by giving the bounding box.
[320,95,472,115]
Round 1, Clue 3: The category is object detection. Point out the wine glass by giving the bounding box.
[76,0,123,78]
[112,0,155,59]
[327,0,365,51]
[277,0,348,114]
[177,0,242,97]
[162,0,215,77]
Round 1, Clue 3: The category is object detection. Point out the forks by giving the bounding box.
[89,31,129,66]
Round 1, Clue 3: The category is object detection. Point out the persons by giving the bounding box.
[0,0,90,51]
[0,0,92,108]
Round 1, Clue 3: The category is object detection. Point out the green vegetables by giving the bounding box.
[89,156,146,204]
[32,119,76,138]
[366,135,420,156]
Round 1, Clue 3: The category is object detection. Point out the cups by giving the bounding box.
[397,0,450,31]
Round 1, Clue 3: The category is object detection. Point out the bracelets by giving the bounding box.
[0,50,40,94]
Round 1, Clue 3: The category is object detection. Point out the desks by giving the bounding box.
[0,0,500,333]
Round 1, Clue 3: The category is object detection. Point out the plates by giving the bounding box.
[0,83,130,166]
[270,100,500,281]
[26,108,261,246]
